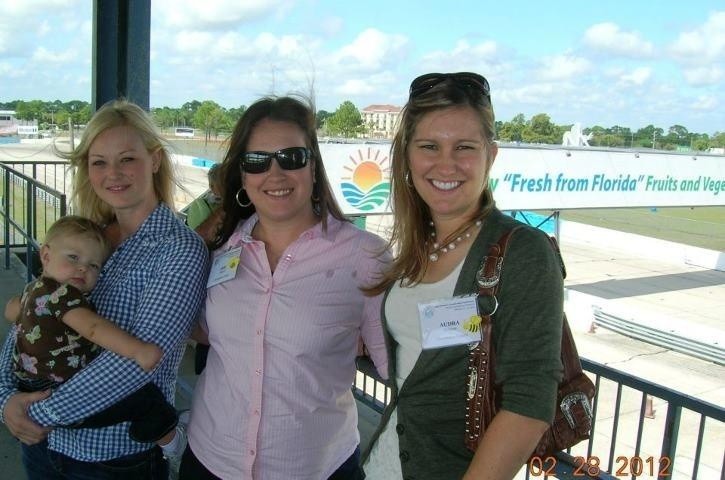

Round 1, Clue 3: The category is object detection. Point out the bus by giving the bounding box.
[175,127,194,137]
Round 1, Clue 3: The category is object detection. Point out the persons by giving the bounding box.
[198,164,243,241]
[0,100,212,480]
[4,216,189,474]
[360,72,567,479]
[174,94,402,479]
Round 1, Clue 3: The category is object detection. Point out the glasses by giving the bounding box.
[409,71,492,96]
[234,145,316,173]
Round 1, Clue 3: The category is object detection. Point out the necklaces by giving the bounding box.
[427,217,484,261]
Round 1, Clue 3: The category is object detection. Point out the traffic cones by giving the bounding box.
[644,395,656,419]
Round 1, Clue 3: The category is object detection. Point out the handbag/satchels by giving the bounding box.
[466,223,595,463]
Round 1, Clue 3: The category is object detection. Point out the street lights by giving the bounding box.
[49,109,55,130]
[651,130,659,149]
